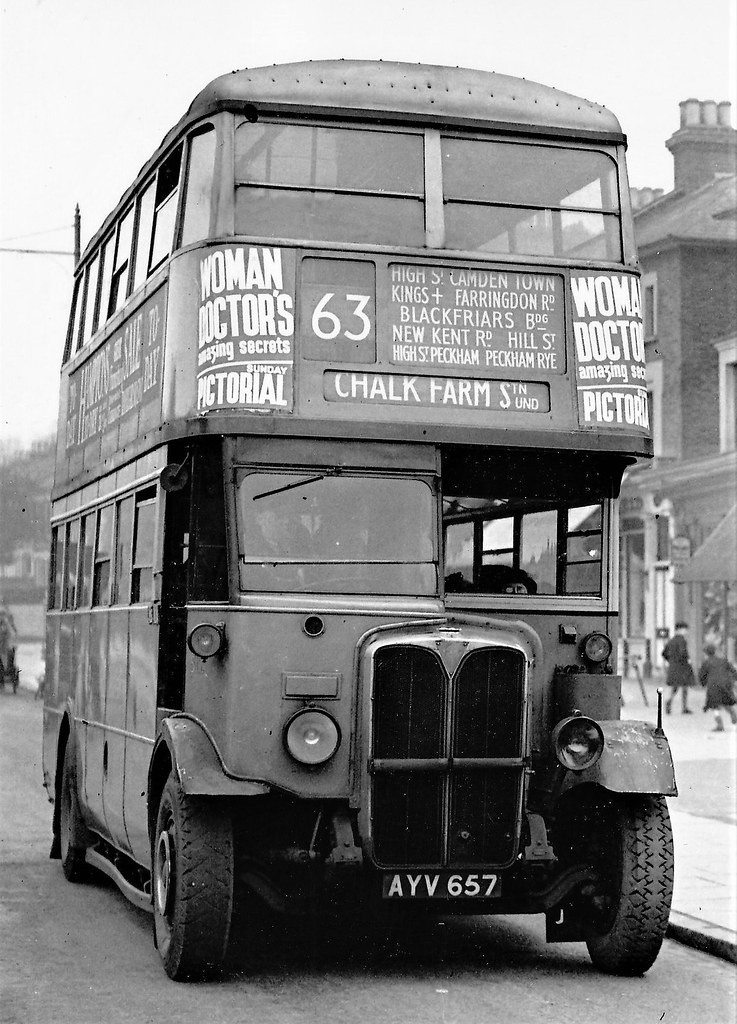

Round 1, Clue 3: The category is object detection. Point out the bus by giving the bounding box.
[44,57,680,985]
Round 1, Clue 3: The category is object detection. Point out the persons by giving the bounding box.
[698,645,737,731]
[472,564,536,597]
[662,623,696,713]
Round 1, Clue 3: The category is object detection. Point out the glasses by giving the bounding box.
[503,586,525,594]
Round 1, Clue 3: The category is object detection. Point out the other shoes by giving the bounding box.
[683,709,691,713]
[666,702,671,714]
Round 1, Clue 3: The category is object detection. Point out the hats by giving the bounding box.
[489,569,537,594]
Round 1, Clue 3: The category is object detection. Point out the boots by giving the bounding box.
[711,716,724,732]
[731,711,737,724]
